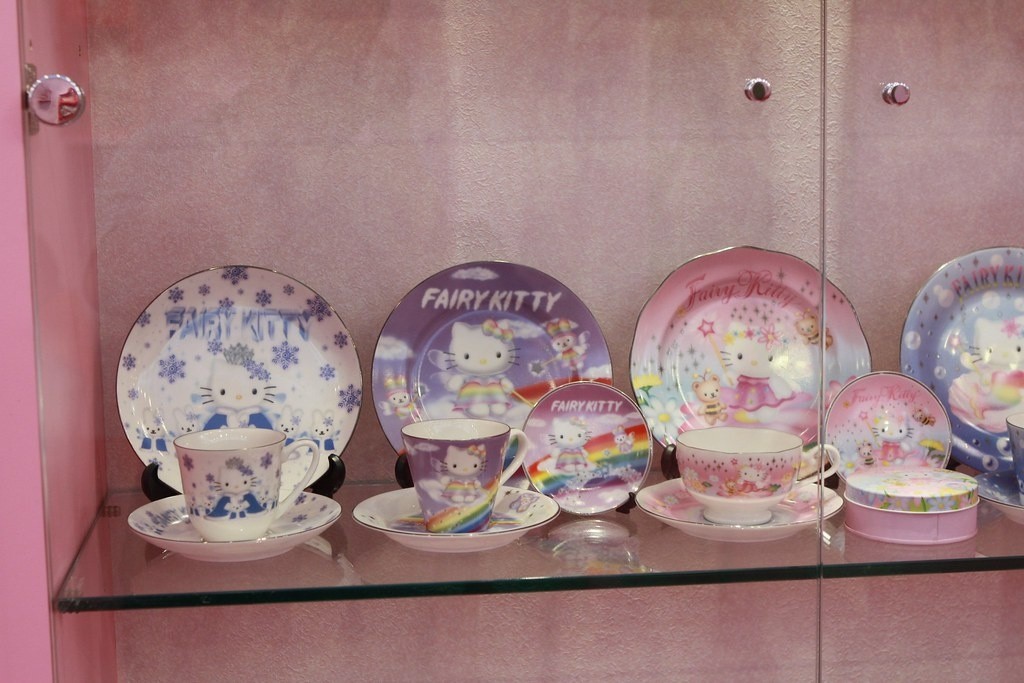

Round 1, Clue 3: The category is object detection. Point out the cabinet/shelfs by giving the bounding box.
[15,0,1024,683]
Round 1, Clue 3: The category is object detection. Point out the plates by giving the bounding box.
[629,245,871,481]
[521,381,653,516]
[351,486,561,553]
[900,245,1024,473]
[973,471,1024,525]
[636,478,844,543]
[371,261,614,492]
[128,491,342,563]
[116,265,364,492]
[824,370,952,484]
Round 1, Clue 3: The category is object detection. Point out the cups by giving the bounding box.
[400,418,529,534]
[173,428,320,543]
[676,426,840,526]
[1006,413,1024,506]
